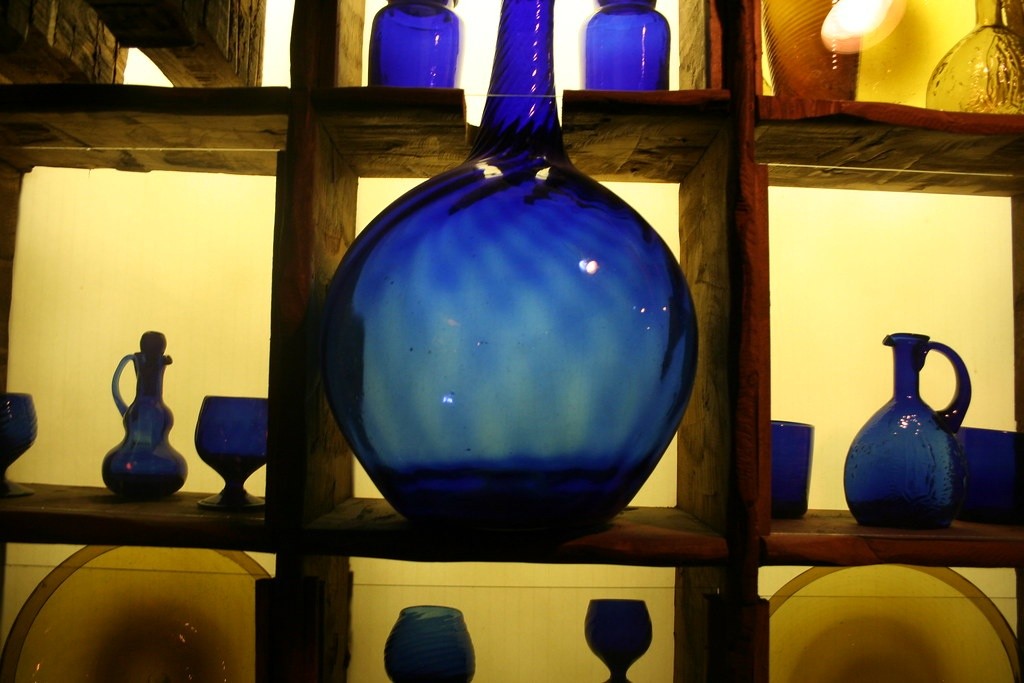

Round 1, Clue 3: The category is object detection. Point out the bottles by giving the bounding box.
[318,0,698,544]
[926,0,1024,115]
[368,0,460,88]
[584,0,670,89]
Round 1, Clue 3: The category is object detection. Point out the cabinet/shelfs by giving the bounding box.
[0,0,1024,683]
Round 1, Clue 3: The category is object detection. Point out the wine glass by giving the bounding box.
[195,396,267,512]
[584,599,652,683]
[0,392,38,499]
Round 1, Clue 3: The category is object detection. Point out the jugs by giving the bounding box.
[844,333,971,529]
[102,331,188,501]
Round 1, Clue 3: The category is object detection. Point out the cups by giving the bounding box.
[955,427,1024,525]
[771,420,814,519]
[384,605,475,683]
